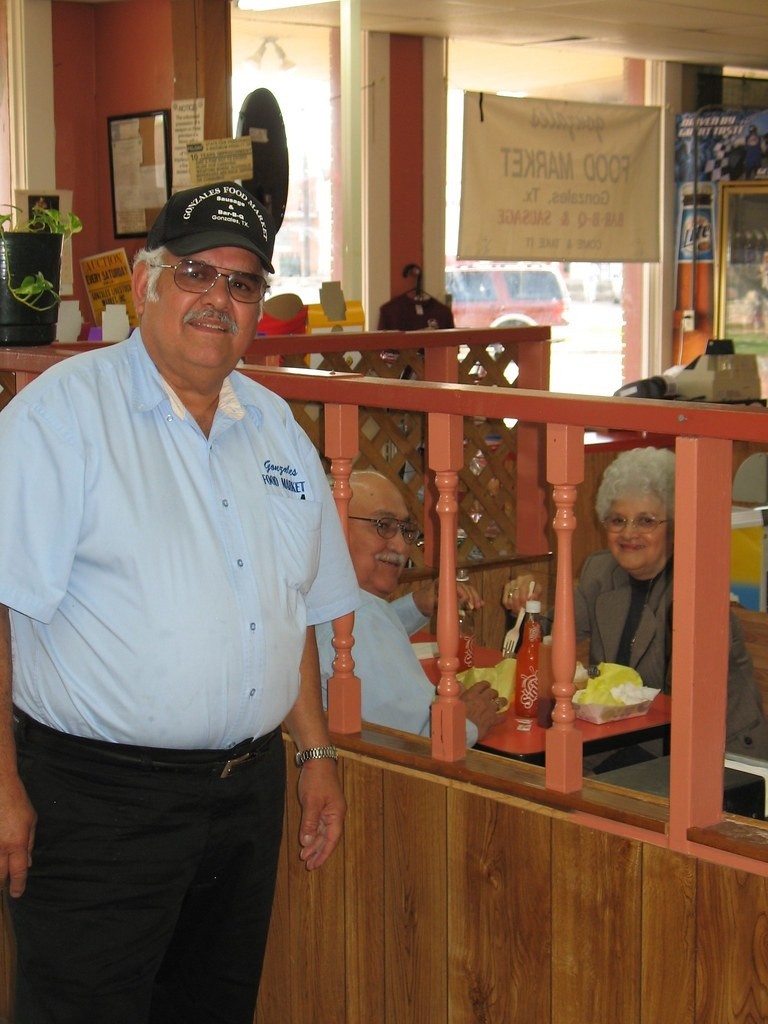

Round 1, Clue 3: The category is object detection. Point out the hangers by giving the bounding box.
[401,263,434,301]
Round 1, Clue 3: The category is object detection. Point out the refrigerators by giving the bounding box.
[671,106,767,376]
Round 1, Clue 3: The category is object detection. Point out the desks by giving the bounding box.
[410,630,672,760]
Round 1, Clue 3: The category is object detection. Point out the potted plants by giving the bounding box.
[0,203,83,346]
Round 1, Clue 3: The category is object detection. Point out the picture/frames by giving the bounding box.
[107,108,172,240]
[711,180,768,353]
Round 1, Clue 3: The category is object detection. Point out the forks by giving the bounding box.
[501,581,534,658]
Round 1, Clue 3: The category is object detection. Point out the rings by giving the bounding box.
[508,592,512,598]
[495,699,502,708]
[513,587,519,590]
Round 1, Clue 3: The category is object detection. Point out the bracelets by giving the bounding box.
[296,745,339,767]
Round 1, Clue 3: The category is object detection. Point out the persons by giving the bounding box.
[315,471,508,746]
[0,181,360,1024]
[502,447,768,787]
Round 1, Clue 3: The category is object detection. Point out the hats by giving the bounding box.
[144,181,275,274]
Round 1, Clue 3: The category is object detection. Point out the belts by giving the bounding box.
[12,716,281,779]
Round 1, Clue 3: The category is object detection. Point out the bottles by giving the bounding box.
[537,636,555,728]
[456,571,474,673]
[727,228,768,331]
[514,602,541,719]
[672,181,714,371]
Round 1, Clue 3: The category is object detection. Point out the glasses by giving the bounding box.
[348,516,421,545]
[600,513,674,534]
[151,259,270,304]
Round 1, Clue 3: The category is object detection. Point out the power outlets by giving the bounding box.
[681,310,695,332]
